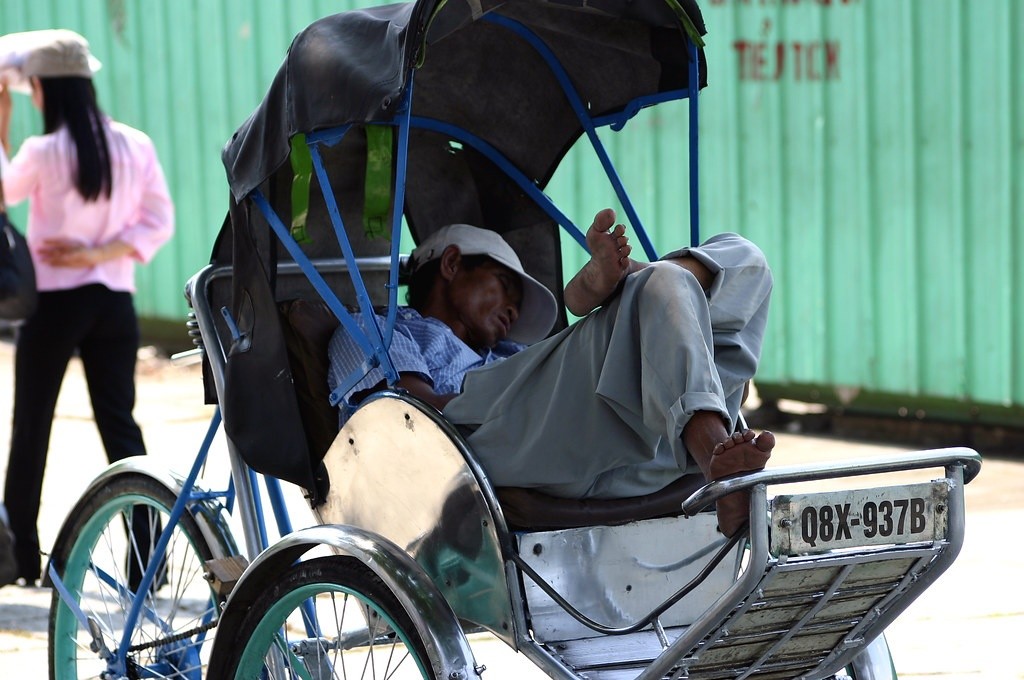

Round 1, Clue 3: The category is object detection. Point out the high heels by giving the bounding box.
[0,571,42,587]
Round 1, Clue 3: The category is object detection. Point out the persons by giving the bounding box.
[330,208,775,539]
[0,32,175,598]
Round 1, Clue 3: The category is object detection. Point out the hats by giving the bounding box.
[407,223,558,346]
[15,29,94,78]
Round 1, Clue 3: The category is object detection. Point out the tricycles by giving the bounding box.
[40,1,986,680]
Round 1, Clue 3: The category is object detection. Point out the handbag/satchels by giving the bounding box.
[0,180,39,321]
